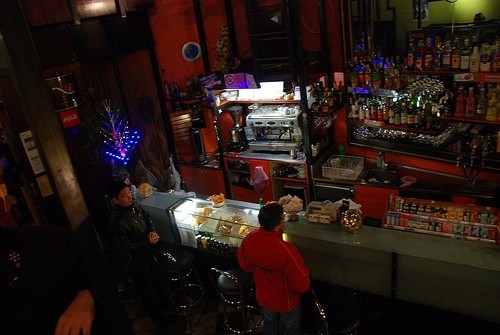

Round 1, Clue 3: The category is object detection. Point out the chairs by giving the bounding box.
[158,248,211,329]
[208,267,264,335]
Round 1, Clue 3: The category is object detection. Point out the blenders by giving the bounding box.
[227,105,249,151]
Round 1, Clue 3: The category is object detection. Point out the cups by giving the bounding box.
[291,149,305,160]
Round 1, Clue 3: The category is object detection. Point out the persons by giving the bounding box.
[0,226,136,335]
[106,180,180,291]
[238,203,310,335]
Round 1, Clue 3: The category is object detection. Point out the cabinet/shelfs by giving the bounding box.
[193,0,335,212]
[341,33,500,174]
[166,194,262,267]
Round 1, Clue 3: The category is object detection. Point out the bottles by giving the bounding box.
[259,198,264,208]
[377,151,384,168]
[163,73,201,119]
[311,80,345,114]
[272,162,306,179]
[195,234,241,258]
[347,32,500,130]
[385,191,497,240]
